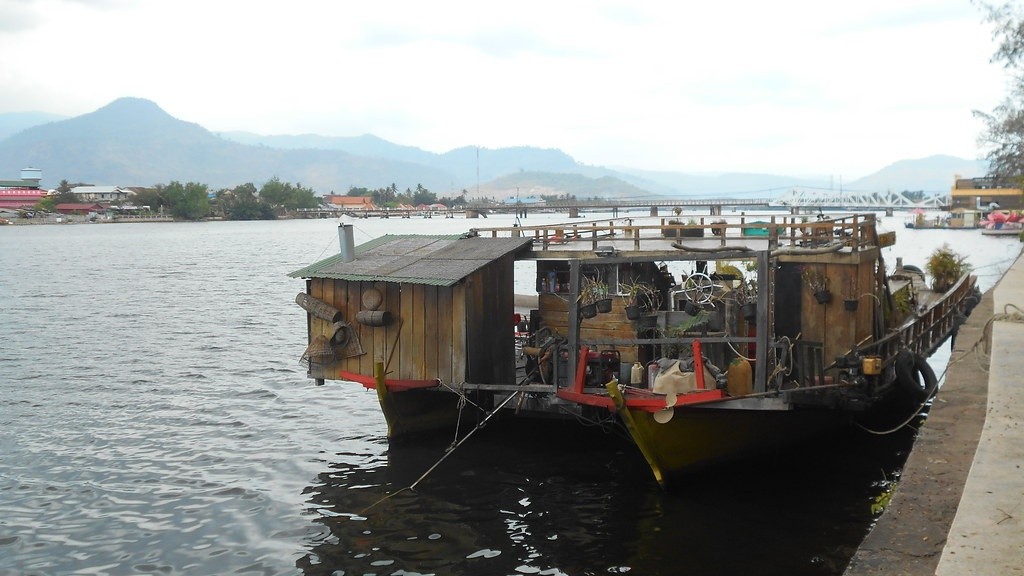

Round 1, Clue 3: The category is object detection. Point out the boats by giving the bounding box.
[981,221,1023,235]
[540,208,555,213]
[903,208,980,230]
[743,221,785,236]
[286,214,980,490]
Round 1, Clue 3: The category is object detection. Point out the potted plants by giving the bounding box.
[737,278,758,321]
[844,291,882,312]
[663,208,705,238]
[618,275,659,327]
[926,247,972,293]
[575,281,612,319]
[711,220,727,235]
[684,280,704,317]
[800,265,832,305]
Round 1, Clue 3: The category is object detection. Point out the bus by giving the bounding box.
[973,176,1013,189]
